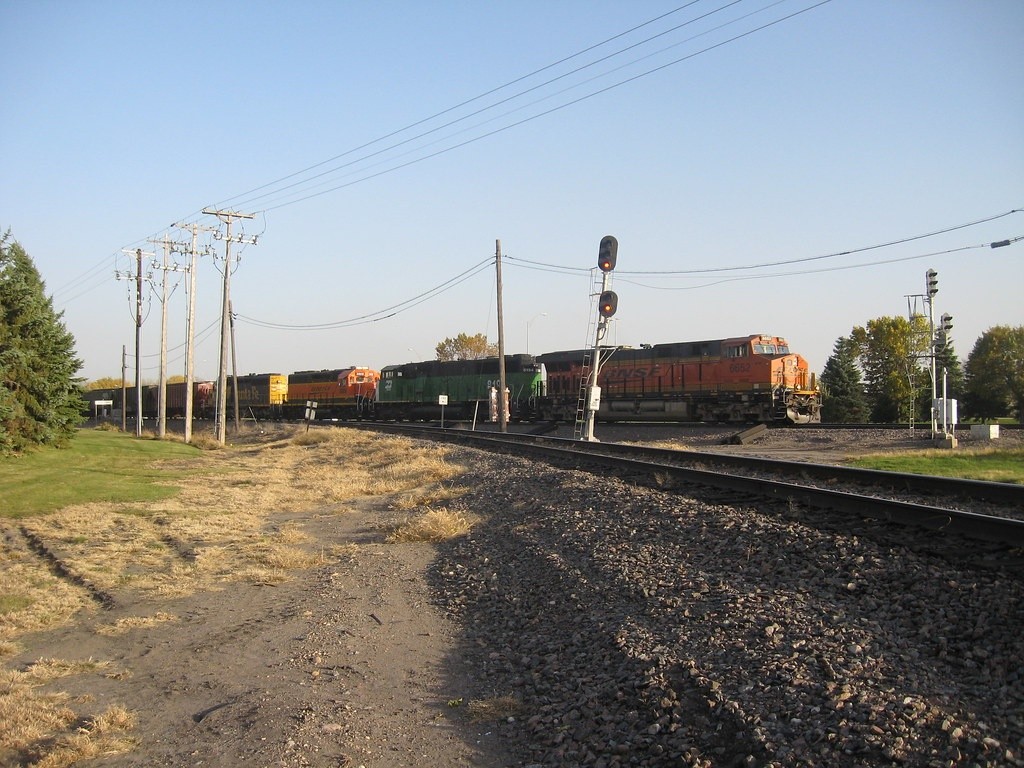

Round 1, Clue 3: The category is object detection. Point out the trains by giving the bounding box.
[68,334,825,425]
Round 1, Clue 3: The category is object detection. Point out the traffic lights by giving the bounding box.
[598,235,618,272]
[926,269,938,298]
[598,291,618,318]
[941,313,953,334]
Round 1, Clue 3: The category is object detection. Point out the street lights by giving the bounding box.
[526,313,547,354]
